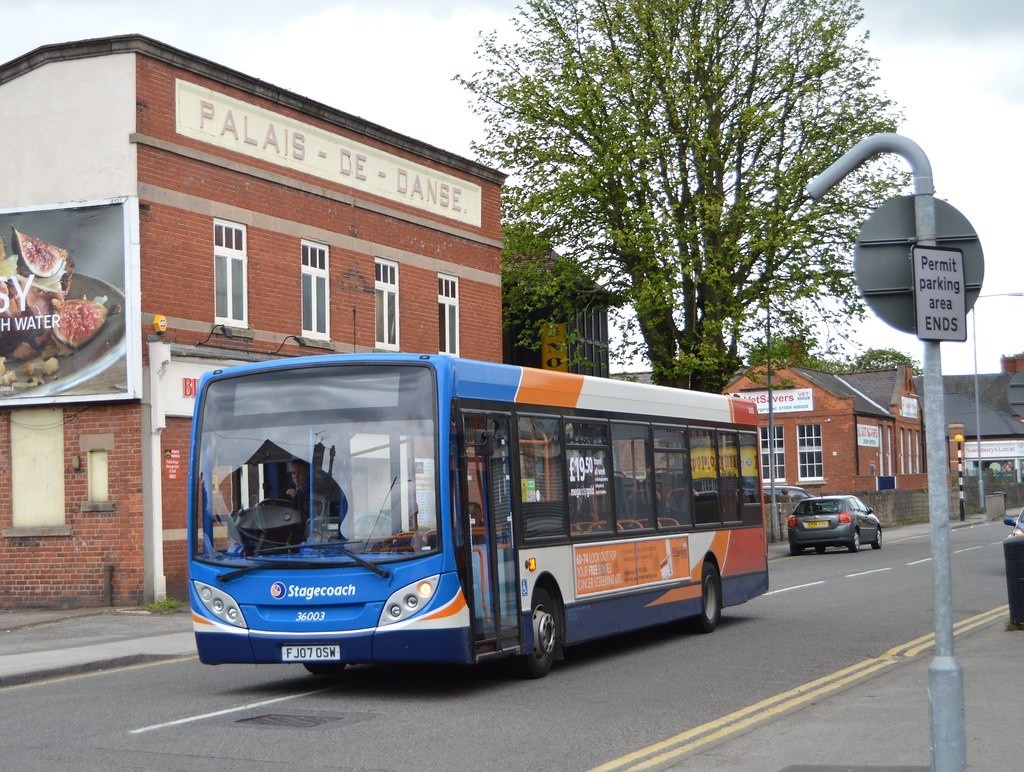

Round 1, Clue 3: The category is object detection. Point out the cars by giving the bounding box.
[1003,507,1024,541]
[764,486,816,498]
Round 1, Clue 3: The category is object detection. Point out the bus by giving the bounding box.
[187,352,769,680]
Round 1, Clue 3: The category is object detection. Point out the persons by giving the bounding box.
[469,502,487,546]
[285,458,322,545]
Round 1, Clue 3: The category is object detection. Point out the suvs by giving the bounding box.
[787,495,882,555]
